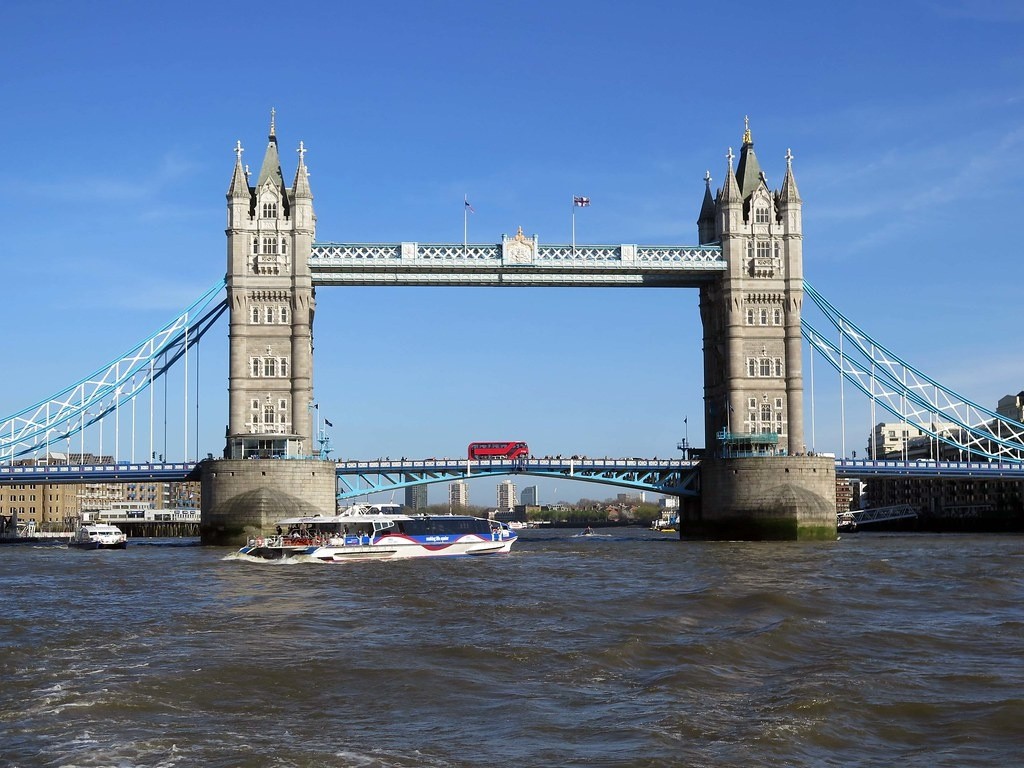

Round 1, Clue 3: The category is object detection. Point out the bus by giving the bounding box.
[466,440,530,460]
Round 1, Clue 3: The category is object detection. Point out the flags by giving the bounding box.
[729,405,735,411]
[465,200,474,214]
[574,196,591,206]
[684,418,688,423]
[325,420,333,427]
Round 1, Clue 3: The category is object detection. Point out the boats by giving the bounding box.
[837,514,856,533]
[67,521,129,549]
[570,528,612,537]
[220,502,519,565]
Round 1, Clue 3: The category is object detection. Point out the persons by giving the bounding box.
[376,455,407,461]
[276,523,374,544]
[530,455,585,459]
[789,450,821,457]
